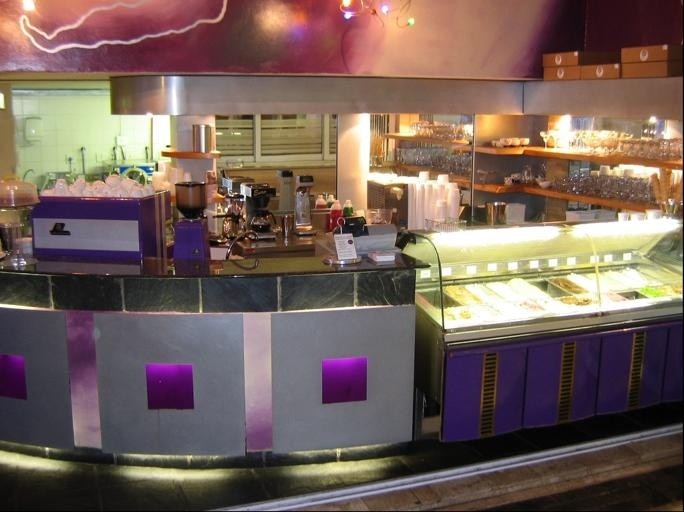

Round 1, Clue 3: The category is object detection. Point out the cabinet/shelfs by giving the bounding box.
[384,115,471,222]
[473,112,683,226]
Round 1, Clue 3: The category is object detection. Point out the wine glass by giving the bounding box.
[540,130,561,151]
[398,120,472,174]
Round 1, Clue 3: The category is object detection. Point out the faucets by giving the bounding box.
[22,169,35,182]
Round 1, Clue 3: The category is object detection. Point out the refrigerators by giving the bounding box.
[402,218,684,444]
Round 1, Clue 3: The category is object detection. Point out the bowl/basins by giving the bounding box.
[511,173,521,180]
[539,181,551,189]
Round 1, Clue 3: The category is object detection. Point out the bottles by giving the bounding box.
[193,124,211,153]
[330,199,353,231]
[316,192,334,207]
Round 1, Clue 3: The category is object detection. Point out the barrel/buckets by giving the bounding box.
[176,182,206,218]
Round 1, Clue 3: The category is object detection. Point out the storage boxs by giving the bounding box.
[541,44,683,80]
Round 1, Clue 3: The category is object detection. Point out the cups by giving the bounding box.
[282,213,292,236]
[504,177,512,185]
[521,166,533,185]
[408,171,460,230]
[492,137,530,148]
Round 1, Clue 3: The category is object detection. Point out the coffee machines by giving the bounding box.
[222,175,254,240]
[240,182,277,241]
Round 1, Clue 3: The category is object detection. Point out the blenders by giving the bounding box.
[172,180,208,260]
